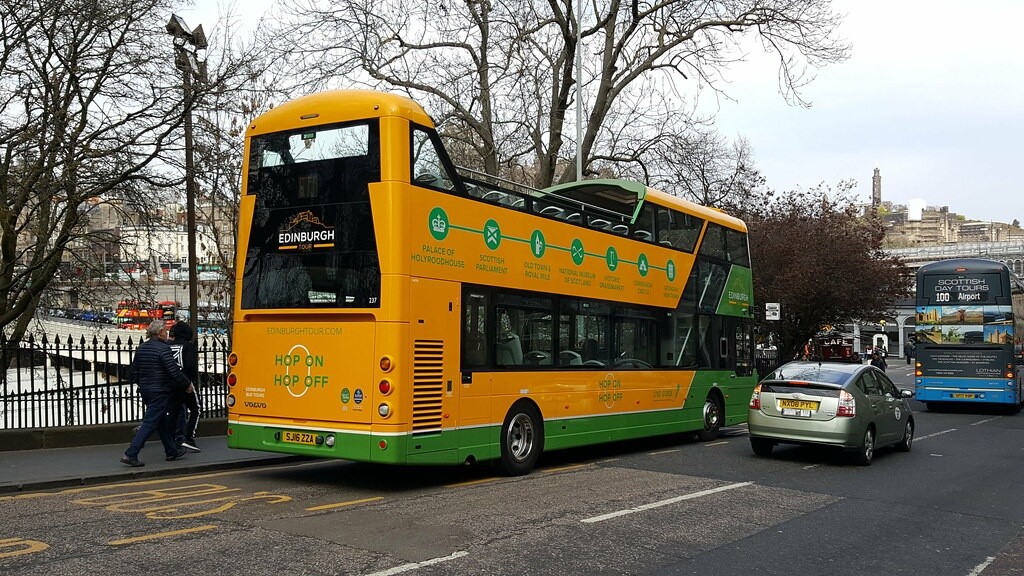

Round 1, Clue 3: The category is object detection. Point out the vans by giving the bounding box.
[964,331,983,344]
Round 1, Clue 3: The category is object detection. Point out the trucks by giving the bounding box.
[807,324,855,363]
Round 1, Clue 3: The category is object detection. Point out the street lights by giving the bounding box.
[165,12,210,349]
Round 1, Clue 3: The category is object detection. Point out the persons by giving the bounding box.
[850,352,862,364]
[165,321,201,451]
[581,339,600,362]
[866,345,888,358]
[905,342,913,364]
[120,320,192,466]
[799,354,809,361]
[871,353,885,372]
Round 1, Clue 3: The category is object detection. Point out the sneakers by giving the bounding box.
[181,438,200,451]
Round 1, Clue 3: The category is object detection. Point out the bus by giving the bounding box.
[116,300,176,331]
[197,264,229,281]
[914,259,1024,415]
[12,266,61,285]
[176,302,226,336]
[225,88,767,478]
[117,262,182,281]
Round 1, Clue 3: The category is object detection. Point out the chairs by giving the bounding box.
[466,331,658,370]
[416,172,675,247]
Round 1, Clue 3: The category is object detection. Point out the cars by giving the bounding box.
[984,311,995,322]
[748,361,916,467]
[994,313,1014,322]
[37,306,117,324]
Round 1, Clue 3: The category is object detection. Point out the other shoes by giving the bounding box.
[119,453,145,467]
[165,448,187,461]
